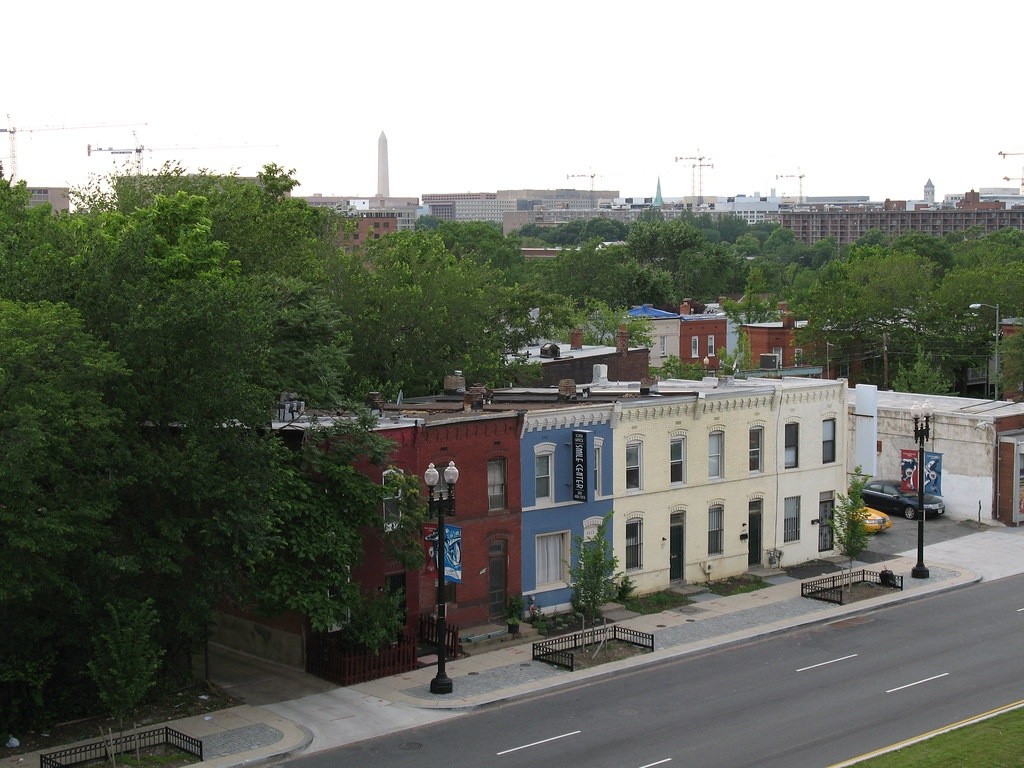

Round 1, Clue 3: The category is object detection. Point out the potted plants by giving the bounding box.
[506,617,523,633]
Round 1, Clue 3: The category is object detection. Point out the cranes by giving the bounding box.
[0,112,149,187]
[87,142,146,196]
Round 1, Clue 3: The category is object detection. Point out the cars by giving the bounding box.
[848,478,946,521]
[848,504,893,536]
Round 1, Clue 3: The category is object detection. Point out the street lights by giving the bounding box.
[969,303,999,401]
[425,460,459,693]
[910,398,933,579]
